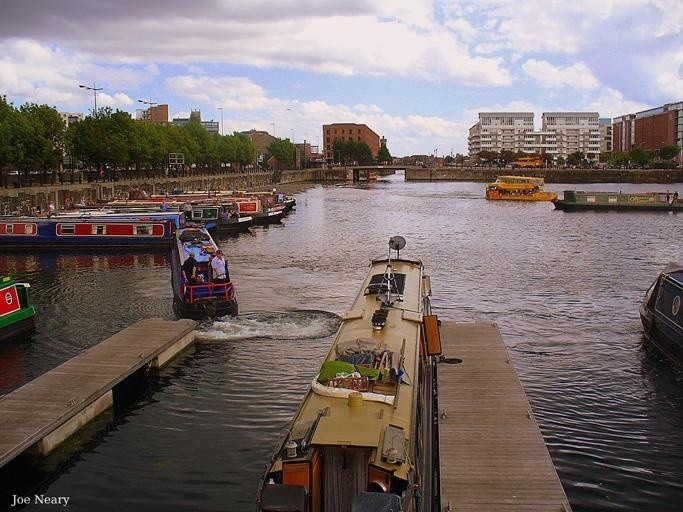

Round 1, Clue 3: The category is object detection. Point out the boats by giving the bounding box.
[171,226,238,320]
[254,258,430,511]
[1,274,36,337]
[486,176,556,200]
[0,187,295,246]
[552,191,682,211]
[638,260,683,368]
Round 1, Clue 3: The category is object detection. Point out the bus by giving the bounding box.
[510,157,541,168]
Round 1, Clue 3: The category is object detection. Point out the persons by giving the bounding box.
[181,252,201,282]
[666,190,678,204]
[184,199,192,222]
[267,199,274,208]
[160,200,169,212]
[208,250,226,284]
[223,209,240,219]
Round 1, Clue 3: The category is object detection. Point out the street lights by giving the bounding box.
[78,84,102,116]
[138,98,157,120]
[217,107,223,137]
